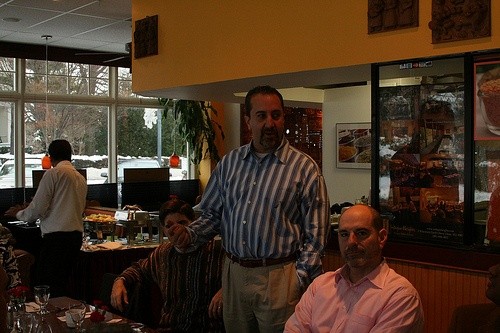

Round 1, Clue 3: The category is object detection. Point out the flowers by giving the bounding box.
[9,284,28,304]
[90,301,108,324]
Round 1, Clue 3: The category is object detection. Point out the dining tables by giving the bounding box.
[78,239,161,297]
[37,296,163,333]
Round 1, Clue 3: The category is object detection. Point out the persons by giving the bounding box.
[167,85,330,333]
[0,222,29,308]
[110,194,226,333]
[4,139,88,298]
[282,204,425,333]
[449,260,500,333]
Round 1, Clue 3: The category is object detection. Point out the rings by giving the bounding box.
[217,306,220,309]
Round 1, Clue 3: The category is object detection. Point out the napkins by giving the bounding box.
[25,301,40,313]
[57,314,91,322]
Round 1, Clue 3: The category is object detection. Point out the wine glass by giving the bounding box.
[34,285,51,315]
[68,303,86,333]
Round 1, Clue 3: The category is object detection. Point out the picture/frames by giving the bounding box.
[336,122,371,170]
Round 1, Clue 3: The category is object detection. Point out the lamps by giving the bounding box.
[42,35,51,170]
[170,99,180,167]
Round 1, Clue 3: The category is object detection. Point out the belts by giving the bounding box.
[225,250,298,268]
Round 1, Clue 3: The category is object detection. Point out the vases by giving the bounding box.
[13,302,27,333]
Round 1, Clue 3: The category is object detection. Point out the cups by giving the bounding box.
[16,313,33,333]
[65,310,76,327]
